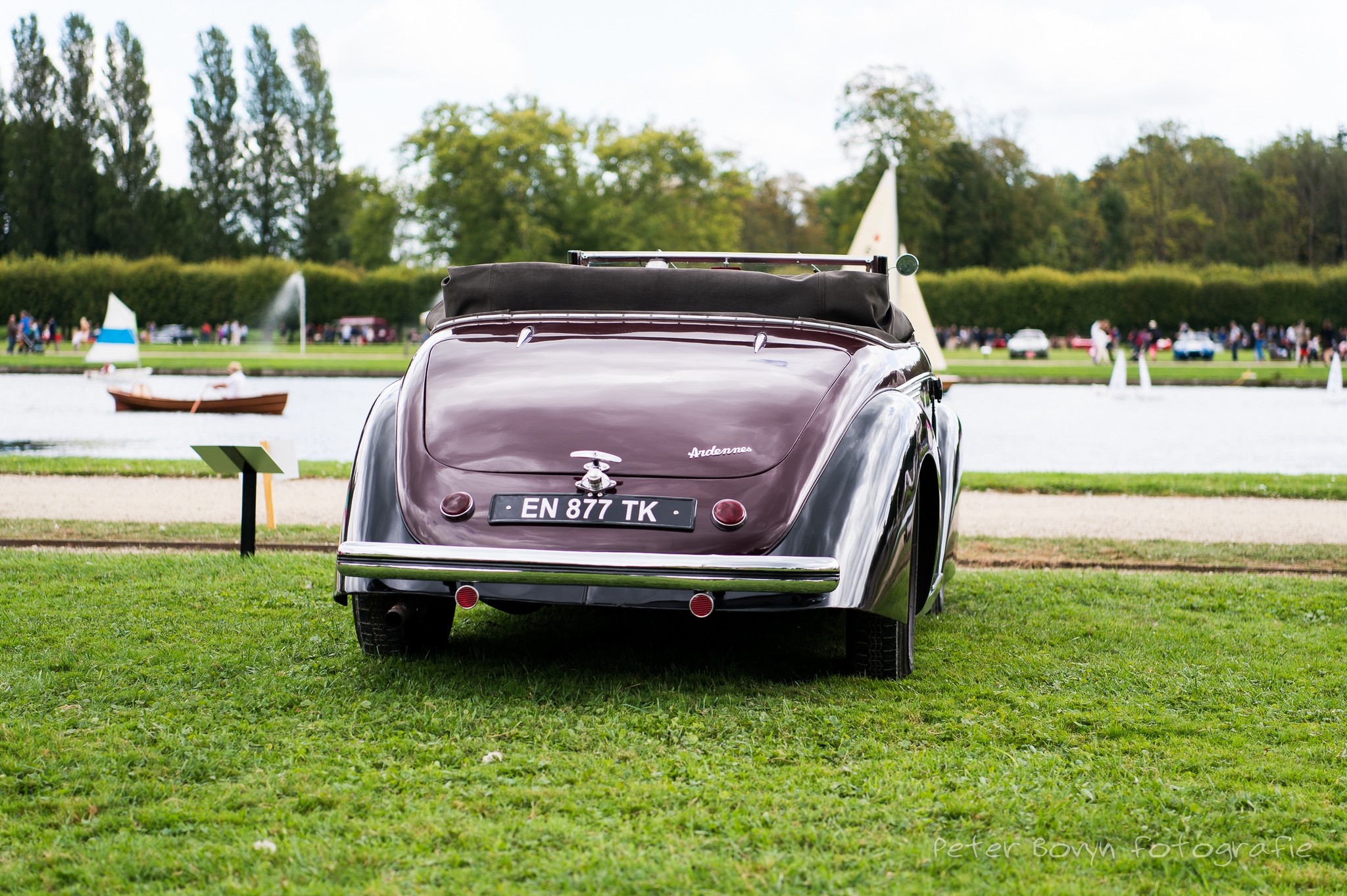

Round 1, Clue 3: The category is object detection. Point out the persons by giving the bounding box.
[5,310,62,356]
[1126,320,1158,362]
[1174,319,1347,368]
[278,319,288,344]
[1088,319,1122,366]
[306,322,352,345]
[933,323,1011,350]
[217,320,248,346]
[138,322,158,344]
[72,316,93,352]
[411,328,430,346]
[357,324,368,345]
[202,323,211,344]
[1050,333,1080,351]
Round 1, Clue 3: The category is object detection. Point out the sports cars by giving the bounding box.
[333,252,963,680]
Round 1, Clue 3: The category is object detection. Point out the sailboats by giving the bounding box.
[82,291,154,385]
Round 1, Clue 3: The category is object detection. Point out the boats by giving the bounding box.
[105,386,288,416]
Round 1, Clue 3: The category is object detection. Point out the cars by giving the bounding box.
[150,324,203,347]
[1007,329,1051,360]
[1172,331,1215,361]
